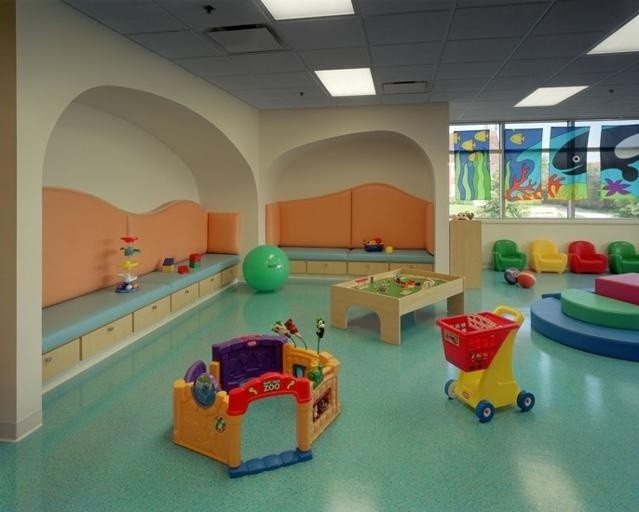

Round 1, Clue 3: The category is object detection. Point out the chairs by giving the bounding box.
[492,240,639,274]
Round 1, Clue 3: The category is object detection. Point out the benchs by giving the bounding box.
[42,253,242,396]
[278,246,435,276]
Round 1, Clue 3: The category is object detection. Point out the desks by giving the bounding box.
[331,267,465,344]
